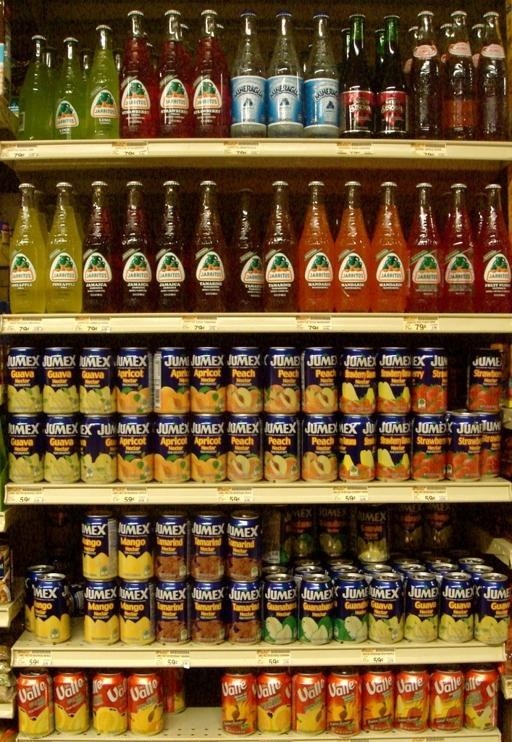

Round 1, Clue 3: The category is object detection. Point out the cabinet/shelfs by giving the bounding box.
[0,132,512,742]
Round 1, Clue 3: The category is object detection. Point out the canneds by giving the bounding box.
[219,666,501,735]
[17,667,187,736]
[6,341,511,413]
[1,506,512,646]
[7,411,505,481]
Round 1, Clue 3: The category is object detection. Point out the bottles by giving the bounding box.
[16,6,505,143]
[0,180,511,313]
